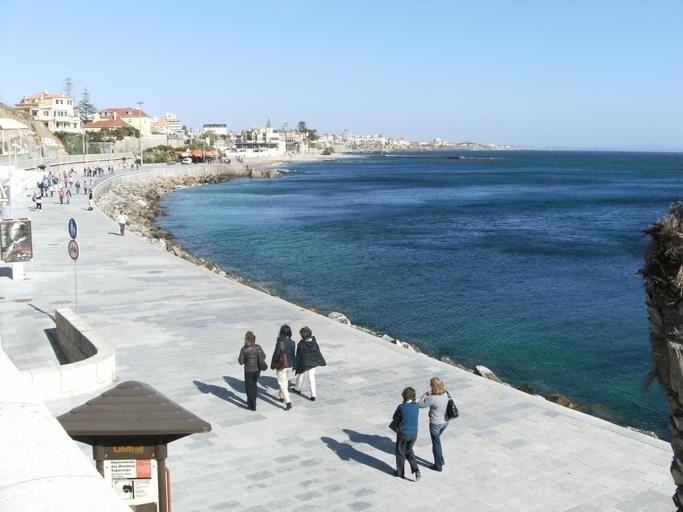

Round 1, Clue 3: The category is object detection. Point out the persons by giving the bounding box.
[392,387,423,482]
[269,324,295,410]
[291,326,327,400]
[25,161,142,210]
[417,376,452,472]
[4,221,30,260]
[118,211,128,235]
[237,331,266,411]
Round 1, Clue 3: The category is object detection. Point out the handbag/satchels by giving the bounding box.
[447,398,459,418]
[273,352,289,369]
[258,352,268,370]
[389,414,404,434]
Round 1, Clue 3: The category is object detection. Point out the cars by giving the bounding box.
[220,156,231,164]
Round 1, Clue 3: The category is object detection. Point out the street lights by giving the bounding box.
[135,100,145,166]
[200,120,206,160]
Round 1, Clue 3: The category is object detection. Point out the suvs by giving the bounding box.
[180,158,193,165]
